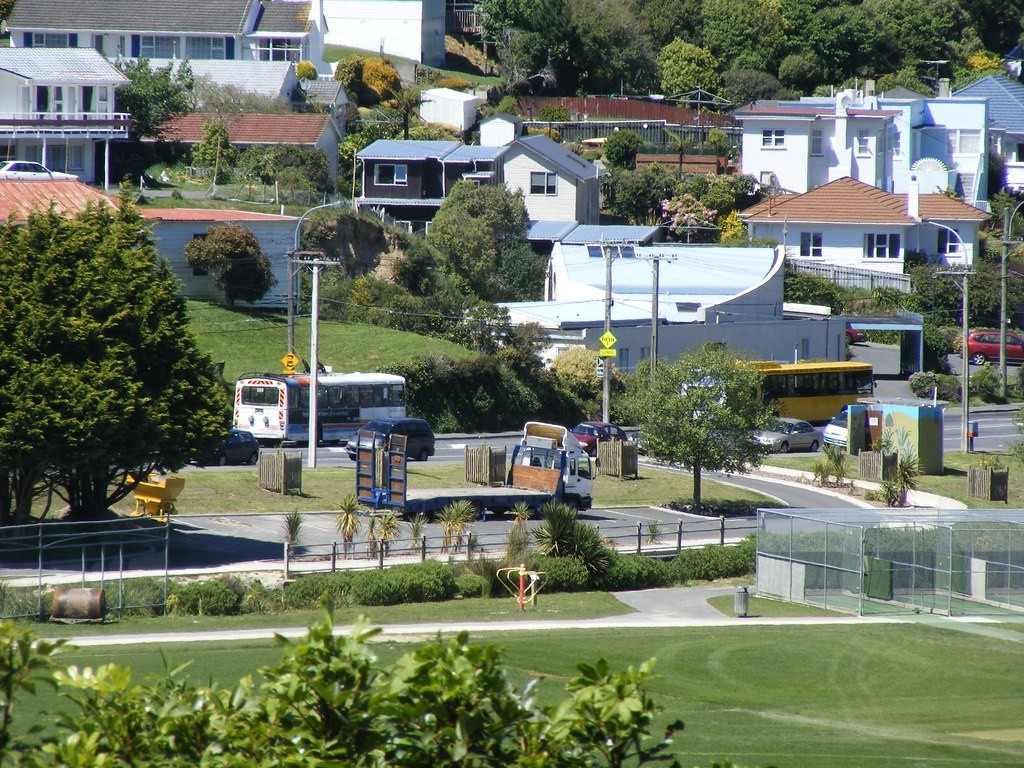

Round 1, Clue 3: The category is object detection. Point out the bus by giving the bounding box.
[679,359,877,431]
[232,372,408,448]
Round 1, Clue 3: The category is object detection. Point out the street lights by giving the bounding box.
[916,218,970,453]
[295,200,345,314]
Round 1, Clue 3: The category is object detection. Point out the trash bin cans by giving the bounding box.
[968,420,978,437]
[734,586,749,617]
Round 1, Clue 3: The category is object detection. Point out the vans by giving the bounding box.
[344,417,436,462]
[824,409,848,451]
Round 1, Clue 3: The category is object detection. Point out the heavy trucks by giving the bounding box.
[355,422,602,525]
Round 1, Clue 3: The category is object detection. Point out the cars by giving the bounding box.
[0,161,80,183]
[628,432,650,455]
[569,421,628,459]
[745,417,824,455]
[846,328,867,346]
[959,329,1024,366]
